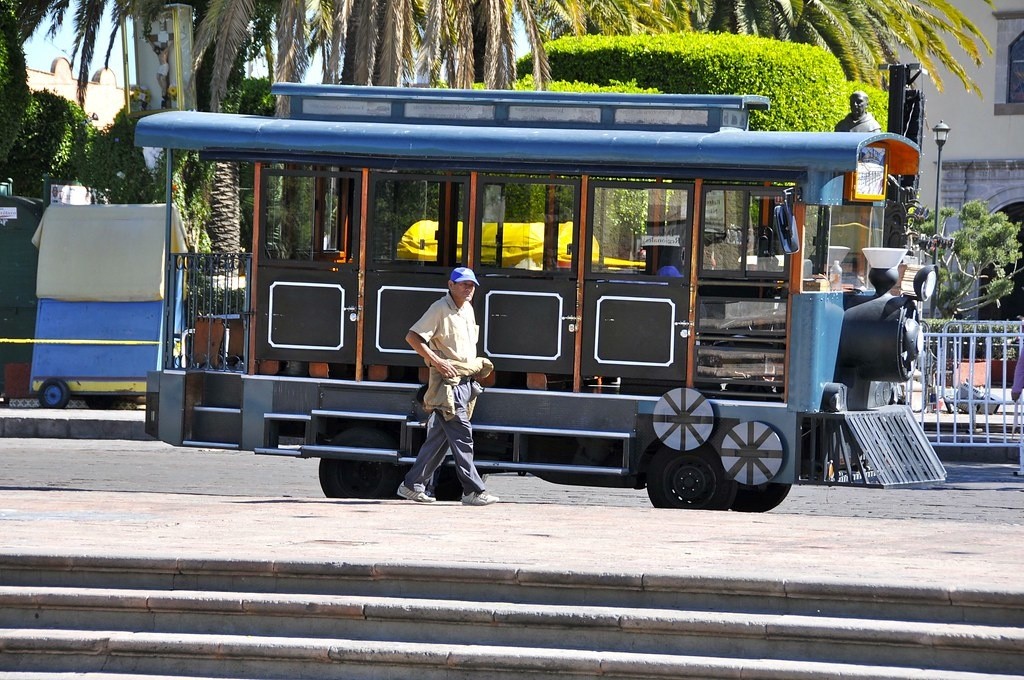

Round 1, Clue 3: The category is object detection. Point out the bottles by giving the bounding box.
[830,260,842,291]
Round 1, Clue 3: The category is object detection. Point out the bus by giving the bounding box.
[132,59,950,513]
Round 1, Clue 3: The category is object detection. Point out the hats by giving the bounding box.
[450,267,479,286]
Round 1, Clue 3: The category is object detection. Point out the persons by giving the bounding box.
[834,90,883,134]
[396,266,499,506]
[1010,341,1024,403]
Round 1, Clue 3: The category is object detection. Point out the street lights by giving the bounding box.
[926,120,952,320]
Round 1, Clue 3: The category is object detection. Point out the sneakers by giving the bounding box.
[462,492,499,506]
[397,481,436,502]
